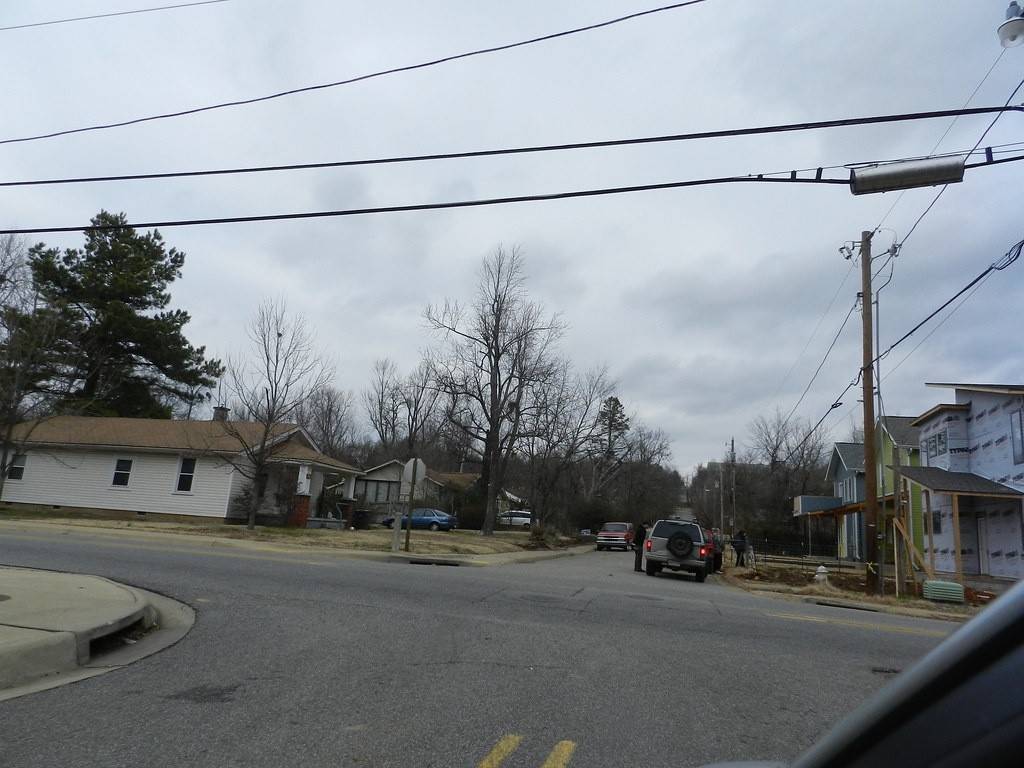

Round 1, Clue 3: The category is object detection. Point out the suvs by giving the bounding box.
[595,521,635,550]
[644,517,709,583]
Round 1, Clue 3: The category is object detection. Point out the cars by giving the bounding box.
[702,526,723,575]
[381,508,456,532]
[496,510,541,530]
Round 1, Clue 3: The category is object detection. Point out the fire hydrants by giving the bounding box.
[813,566,828,591]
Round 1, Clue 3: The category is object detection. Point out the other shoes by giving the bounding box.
[716,570,724,575]
[634,567,645,572]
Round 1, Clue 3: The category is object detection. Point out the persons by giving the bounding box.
[631,520,649,572]
[711,527,724,574]
[733,529,746,566]
[645,524,653,540]
[315,495,323,517]
